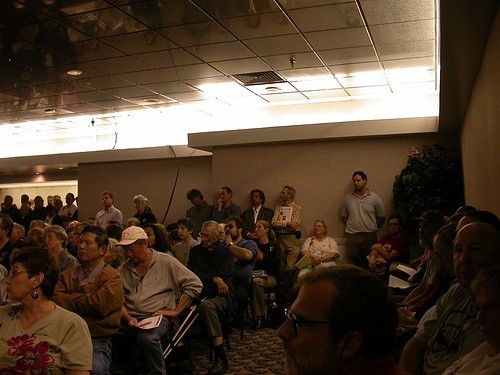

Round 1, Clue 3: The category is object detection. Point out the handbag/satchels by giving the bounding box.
[296,231,302,240]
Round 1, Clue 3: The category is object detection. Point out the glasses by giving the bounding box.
[6,269,32,279]
[224,224,237,229]
[199,232,211,239]
[284,308,333,337]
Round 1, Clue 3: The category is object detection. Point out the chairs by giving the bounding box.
[111,278,280,375]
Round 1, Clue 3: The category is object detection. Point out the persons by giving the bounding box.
[276,264,398,375]
[340,171,385,270]
[384,206,500,375]
[368,216,410,278]
[298,220,339,279]
[0,186,304,375]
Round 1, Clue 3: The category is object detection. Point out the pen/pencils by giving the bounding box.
[136,322,153,328]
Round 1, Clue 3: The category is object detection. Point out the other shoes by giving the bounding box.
[207,358,229,375]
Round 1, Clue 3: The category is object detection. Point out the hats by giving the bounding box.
[115,226,148,246]
[416,209,444,226]
